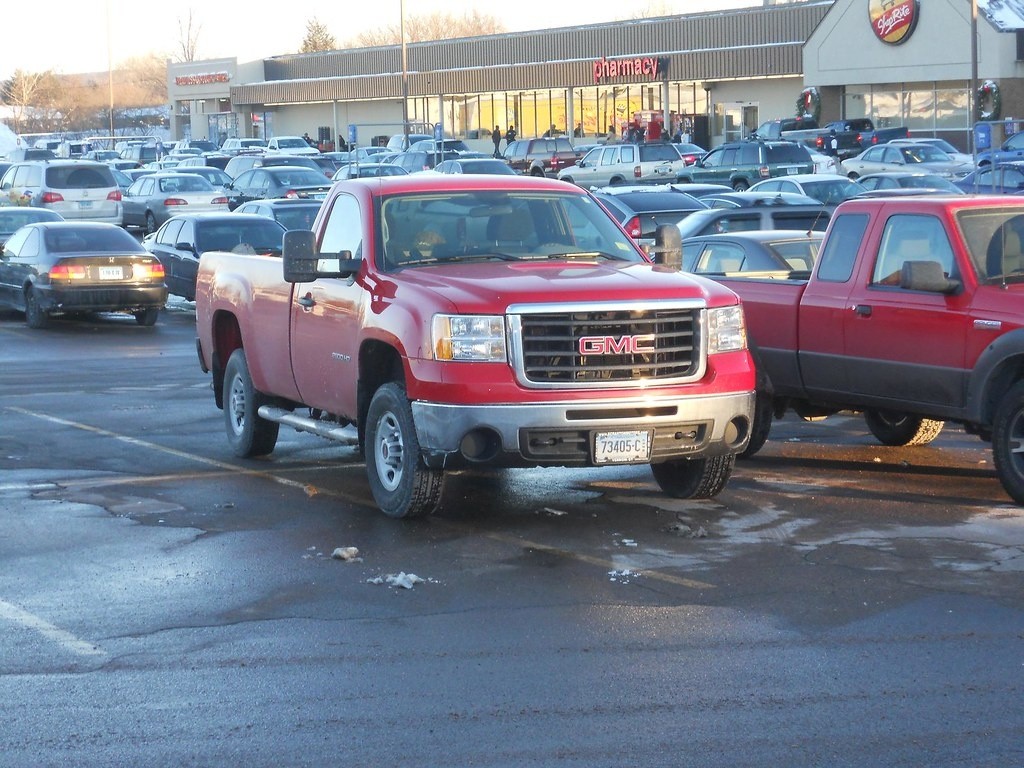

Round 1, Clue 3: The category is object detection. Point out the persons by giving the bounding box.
[575,123,585,137]
[302,133,309,144]
[340,135,344,147]
[825,129,837,156]
[219,132,227,147]
[681,130,689,143]
[607,126,615,141]
[492,126,501,156]
[549,124,556,136]
[506,126,516,144]
[676,128,682,136]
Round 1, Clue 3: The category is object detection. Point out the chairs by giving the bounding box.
[487,208,533,256]
[901,260,961,292]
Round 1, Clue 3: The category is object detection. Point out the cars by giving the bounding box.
[1,119,1024,326]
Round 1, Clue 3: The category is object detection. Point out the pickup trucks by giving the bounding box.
[195,173,758,522]
[696,194,1023,506]
